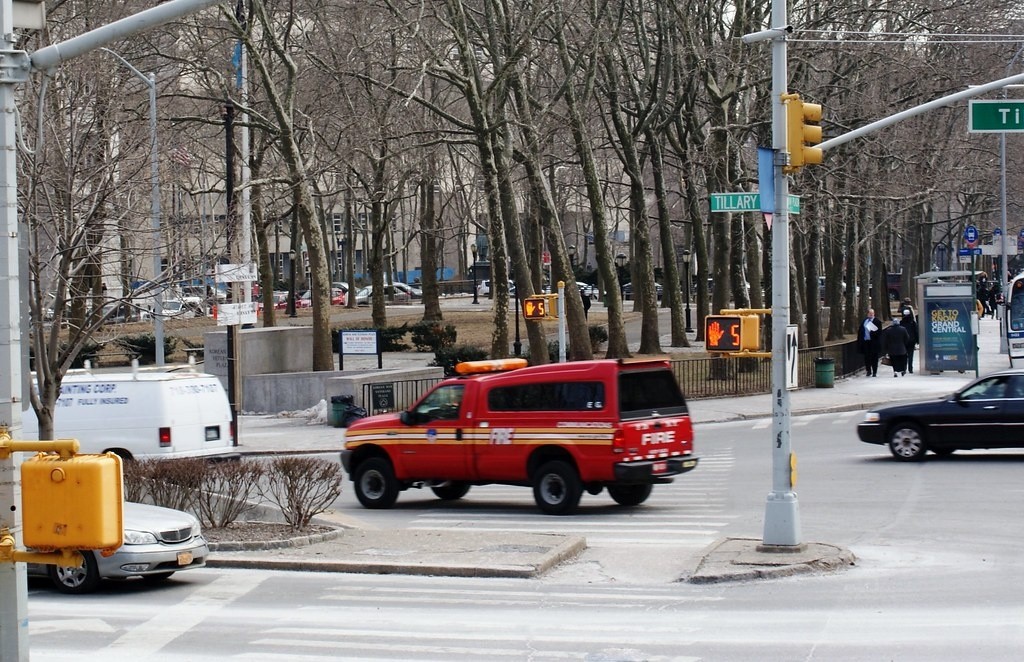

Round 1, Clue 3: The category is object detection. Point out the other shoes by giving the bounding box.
[894,371,906,377]
[866,371,876,377]
[908,367,914,373]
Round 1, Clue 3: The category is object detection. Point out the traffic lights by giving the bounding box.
[787,92,823,171]
[704,315,760,352]
[524,298,546,320]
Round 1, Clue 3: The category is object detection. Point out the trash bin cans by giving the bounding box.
[331,395,353,426]
[815,358,834,388]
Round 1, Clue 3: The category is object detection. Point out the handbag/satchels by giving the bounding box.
[881,358,891,365]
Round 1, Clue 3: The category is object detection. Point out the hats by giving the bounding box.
[580,289,585,292]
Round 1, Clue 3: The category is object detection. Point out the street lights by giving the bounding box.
[288,249,298,318]
[569,245,576,269]
[471,243,480,305]
[616,253,627,301]
[682,249,694,333]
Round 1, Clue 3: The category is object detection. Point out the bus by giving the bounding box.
[995,270,1024,368]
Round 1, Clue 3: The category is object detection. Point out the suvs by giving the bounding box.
[869,273,903,302]
[338,354,700,514]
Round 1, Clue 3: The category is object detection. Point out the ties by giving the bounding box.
[868,318,871,323]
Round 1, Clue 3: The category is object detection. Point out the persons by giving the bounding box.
[858,309,883,377]
[581,290,591,321]
[882,298,920,376]
[977,266,1021,321]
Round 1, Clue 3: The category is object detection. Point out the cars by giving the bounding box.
[27,501,210,592]
[856,368,1024,462]
[817,277,861,299]
[85,279,664,325]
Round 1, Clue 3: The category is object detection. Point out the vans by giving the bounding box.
[22,370,242,467]
